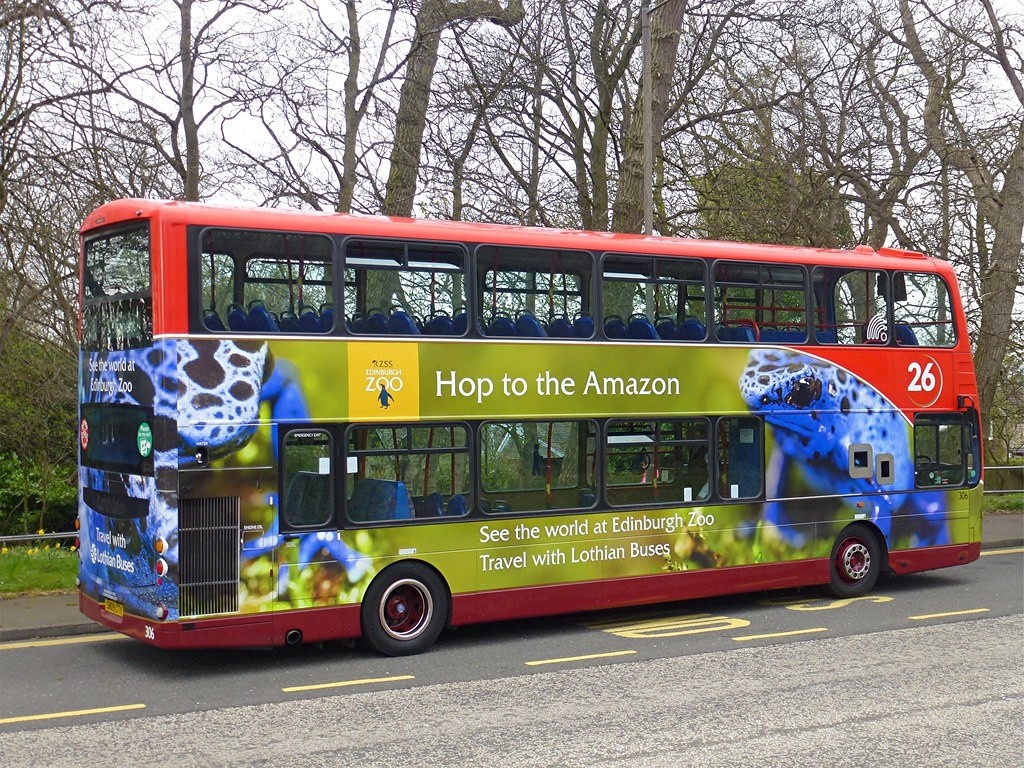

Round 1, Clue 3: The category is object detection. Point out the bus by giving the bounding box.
[76,196,987,657]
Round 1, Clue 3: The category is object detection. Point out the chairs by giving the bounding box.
[203,299,916,529]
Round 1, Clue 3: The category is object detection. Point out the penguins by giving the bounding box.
[377,383,394,409]
